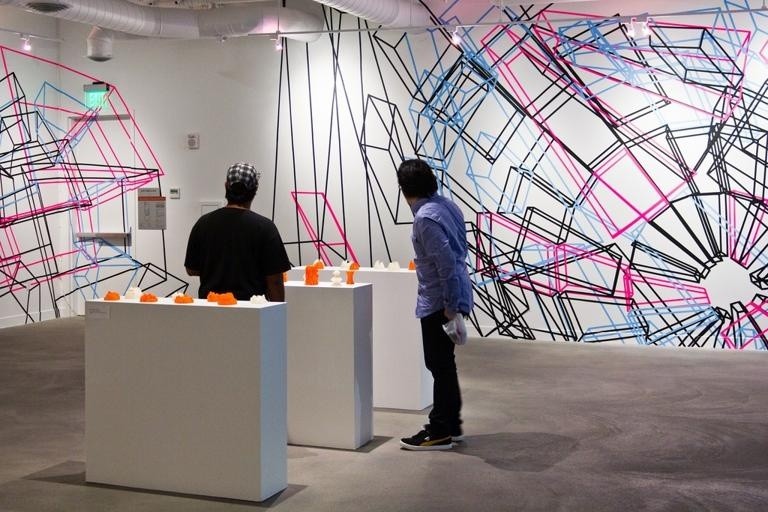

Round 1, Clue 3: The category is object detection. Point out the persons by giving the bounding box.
[184,162,291,304]
[397,157,474,451]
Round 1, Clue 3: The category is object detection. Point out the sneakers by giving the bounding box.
[399,430,452,451]
[423,423,464,441]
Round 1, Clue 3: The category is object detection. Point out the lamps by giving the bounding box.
[20,32,33,51]
[247,5,768,50]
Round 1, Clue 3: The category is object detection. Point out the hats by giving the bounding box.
[226,163,262,190]
